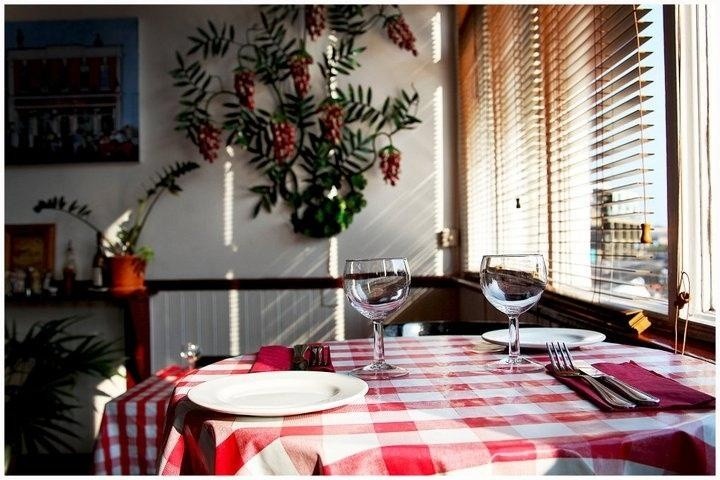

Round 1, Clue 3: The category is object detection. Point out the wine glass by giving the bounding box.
[481,254,548,372]
[341,256,411,380]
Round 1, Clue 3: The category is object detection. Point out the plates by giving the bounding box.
[188,370,369,416]
[482,328,606,349]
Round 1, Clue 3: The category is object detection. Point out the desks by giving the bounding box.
[5,291,158,391]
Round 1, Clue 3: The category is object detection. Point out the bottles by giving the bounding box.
[91,232,108,289]
[63,239,76,282]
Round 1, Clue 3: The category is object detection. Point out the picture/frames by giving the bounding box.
[4,17,139,167]
[5,223,56,273]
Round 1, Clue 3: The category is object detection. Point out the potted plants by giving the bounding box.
[31,160,201,293]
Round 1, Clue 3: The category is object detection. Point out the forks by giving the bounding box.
[546,342,637,410]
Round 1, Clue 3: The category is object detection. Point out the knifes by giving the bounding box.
[292,343,306,370]
[573,359,661,406]
